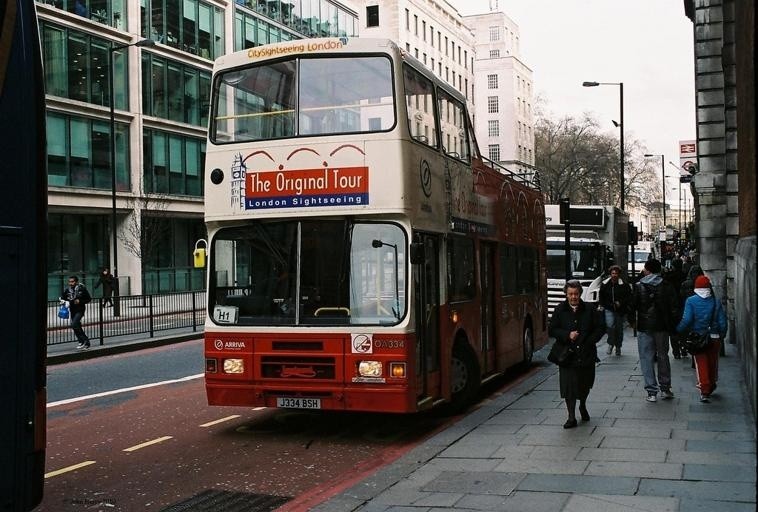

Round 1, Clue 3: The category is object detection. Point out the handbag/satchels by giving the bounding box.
[679,330,712,354]
[110,277,116,291]
[548,340,581,369]
[605,309,616,329]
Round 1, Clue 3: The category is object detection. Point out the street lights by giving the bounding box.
[107,36,162,318]
[642,150,690,251]
[370,238,401,321]
[582,77,631,229]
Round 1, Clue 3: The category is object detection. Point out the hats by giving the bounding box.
[695,275,712,288]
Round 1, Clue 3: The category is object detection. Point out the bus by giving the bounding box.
[0,0,57,501]
[190,38,552,423]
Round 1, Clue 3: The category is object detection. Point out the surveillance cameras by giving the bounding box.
[688,163,699,174]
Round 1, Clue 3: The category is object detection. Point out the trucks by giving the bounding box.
[542,200,631,332]
[627,240,658,282]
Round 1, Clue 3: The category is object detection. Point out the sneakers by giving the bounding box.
[645,396,656,402]
[83,345,90,349]
[660,389,673,399]
[616,347,621,355]
[606,345,614,354]
[76,340,87,349]
[700,395,709,401]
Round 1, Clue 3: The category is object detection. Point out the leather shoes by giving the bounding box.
[564,419,577,428]
[579,406,591,421]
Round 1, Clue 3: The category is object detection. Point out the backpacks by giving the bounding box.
[627,281,655,333]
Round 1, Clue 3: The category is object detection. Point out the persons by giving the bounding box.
[598,232,729,402]
[95,264,115,309]
[57,274,94,349]
[550,281,601,428]
